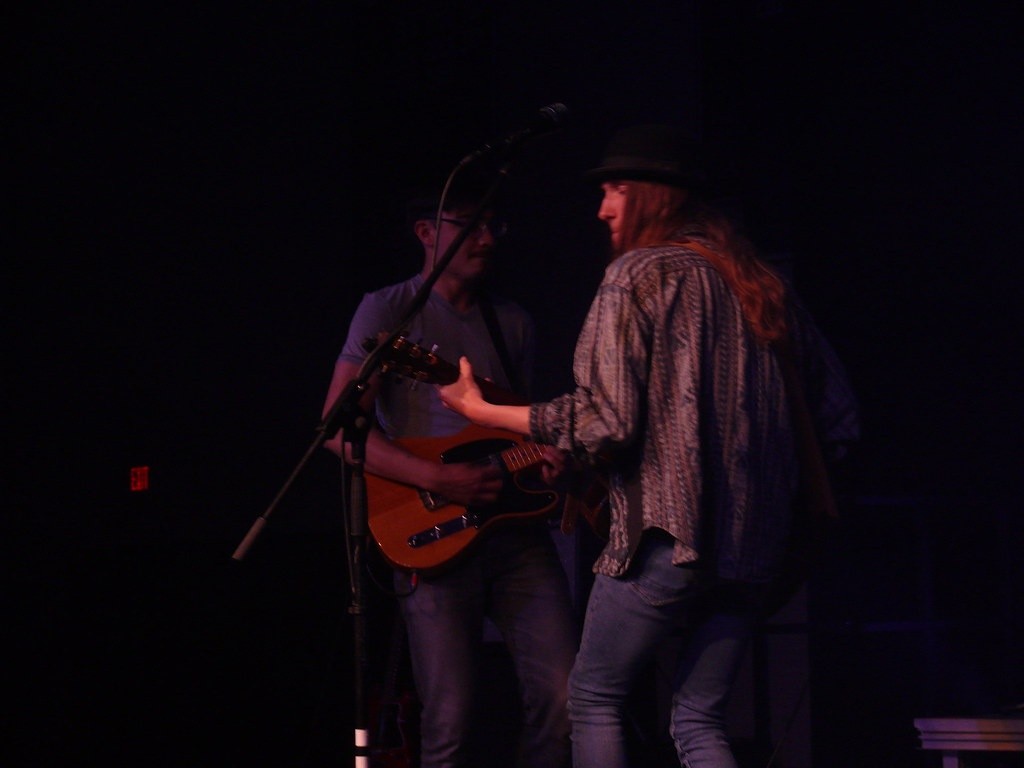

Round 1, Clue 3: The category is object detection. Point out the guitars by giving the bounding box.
[359,400,562,577]
[361,328,526,410]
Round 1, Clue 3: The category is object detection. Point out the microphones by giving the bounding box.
[476,102,570,156]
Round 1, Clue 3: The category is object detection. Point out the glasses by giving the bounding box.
[430,218,507,238]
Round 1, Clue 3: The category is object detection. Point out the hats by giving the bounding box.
[593,126,703,185]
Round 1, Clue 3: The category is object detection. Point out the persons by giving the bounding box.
[320,177,579,768]
[433,127,856,768]
